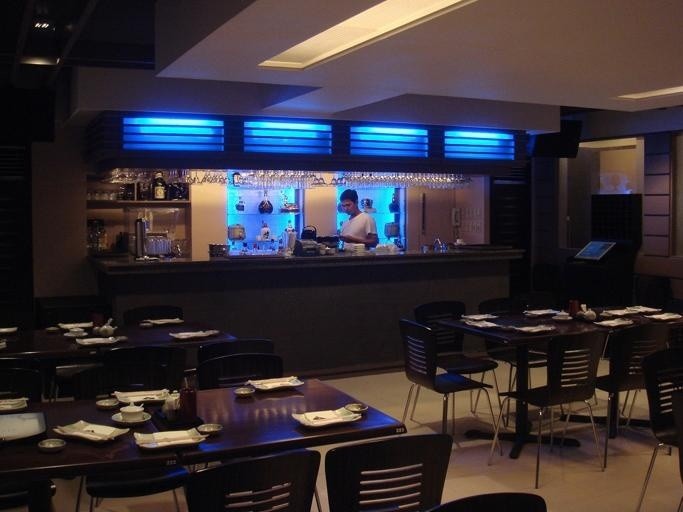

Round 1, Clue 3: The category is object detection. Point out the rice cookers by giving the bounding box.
[227,224,245,240]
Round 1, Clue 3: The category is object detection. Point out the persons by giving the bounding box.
[338,190,378,250]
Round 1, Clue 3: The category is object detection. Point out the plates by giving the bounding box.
[113,389,169,405]
[133,428,206,450]
[0,411,47,442]
[460,306,682,334]
[291,407,362,427]
[57,318,220,346]
[0,398,28,413]
[52,419,130,443]
[111,412,151,426]
[247,376,304,392]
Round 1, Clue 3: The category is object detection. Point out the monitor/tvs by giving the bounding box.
[575,240,616,262]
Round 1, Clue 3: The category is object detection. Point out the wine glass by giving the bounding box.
[167,169,471,189]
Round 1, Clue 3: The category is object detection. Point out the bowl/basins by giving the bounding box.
[94,399,119,410]
[344,403,368,414]
[37,439,65,453]
[234,387,255,398]
[196,423,223,435]
[351,243,364,255]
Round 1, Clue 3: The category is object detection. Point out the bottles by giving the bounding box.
[284,219,294,232]
[234,195,247,214]
[134,218,145,258]
[229,238,284,257]
[258,191,273,214]
[152,170,165,200]
[432,237,442,252]
[260,223,271,241]
[165,386,197,425]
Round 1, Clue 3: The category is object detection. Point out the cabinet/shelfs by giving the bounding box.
[335,208,399,239]
[85,197,192,257]
[226,208,300,243]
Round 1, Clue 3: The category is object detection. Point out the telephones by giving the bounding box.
[451,208,460,227]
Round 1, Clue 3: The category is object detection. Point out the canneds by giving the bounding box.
[179,388,197,423]
[568,300,578,316]
